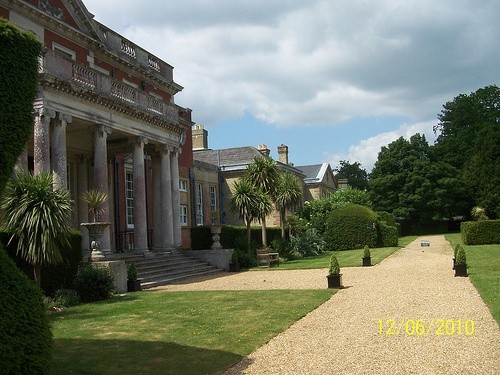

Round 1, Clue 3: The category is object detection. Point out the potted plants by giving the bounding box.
[80,189,110,262]
[452,243,468,277]
[361,245,372,267]
[326,254,343,288]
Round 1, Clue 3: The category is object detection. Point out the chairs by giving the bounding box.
[257,249,279,269]
